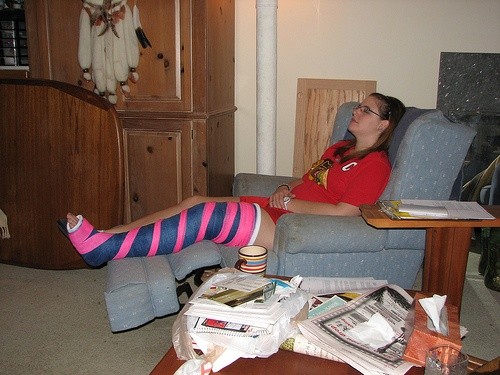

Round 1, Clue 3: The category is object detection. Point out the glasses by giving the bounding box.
[352,102,388,122]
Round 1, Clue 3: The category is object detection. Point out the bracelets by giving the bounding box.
[283,196,290,211]
[277,184,291,191]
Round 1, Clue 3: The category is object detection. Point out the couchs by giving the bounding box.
[103,102,478,333]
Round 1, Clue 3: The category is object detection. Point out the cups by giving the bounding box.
[424,344,469,375]
[234,245,268,278]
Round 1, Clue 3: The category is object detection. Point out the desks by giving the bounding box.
[359,204,500,324]
[148,274,426,375]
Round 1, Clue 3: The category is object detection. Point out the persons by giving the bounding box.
[55,91,408,269]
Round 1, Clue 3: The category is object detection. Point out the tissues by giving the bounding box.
[400,290,463,372]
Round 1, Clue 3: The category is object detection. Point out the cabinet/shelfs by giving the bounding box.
[24,0,237,222]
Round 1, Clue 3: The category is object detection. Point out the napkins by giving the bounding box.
[418,294,447,331]
